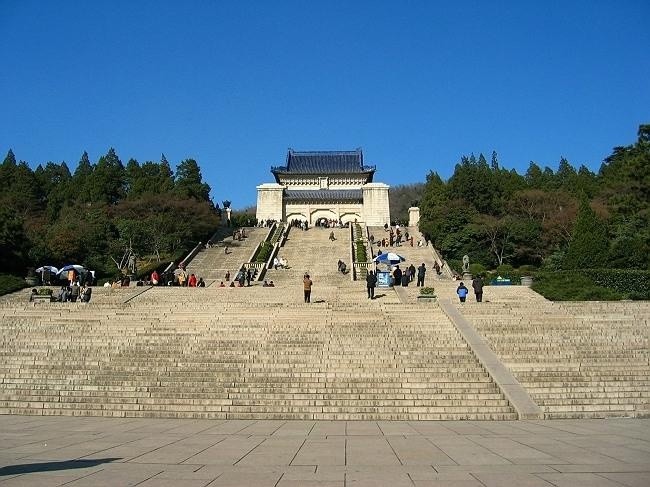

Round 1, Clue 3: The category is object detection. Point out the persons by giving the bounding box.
[240,217,347,231]
[303,275,312,302]
[219,256,290,288]
[102,262,205,287]
[366,270,378,299]
[368,213,431,248]
[338,261,349,275]
[205,226,247,256]
[391,258,441,288]
[227,219,231,228]
[329,230,336,240]
[457,282,468,301]
[336,259,342,271]
[472,275,484,302]
[52,284,92,302]
[29,288,38,301]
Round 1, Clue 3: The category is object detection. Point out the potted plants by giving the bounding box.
[416,287,436,302]
[518,264,534,287]
[31,287,57,303]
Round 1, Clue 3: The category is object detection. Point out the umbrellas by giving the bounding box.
[34,265,58,274]
[372,252,405,266]
[55,263,84,280]
